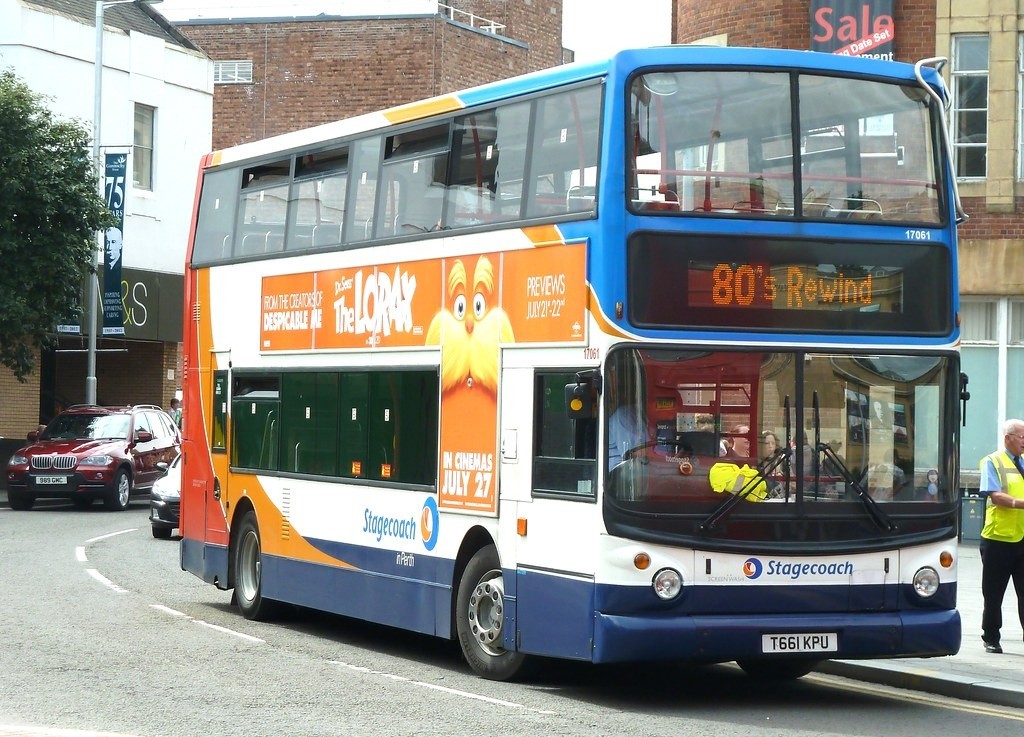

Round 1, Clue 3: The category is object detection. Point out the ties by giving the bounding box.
[1014,456,1023,478]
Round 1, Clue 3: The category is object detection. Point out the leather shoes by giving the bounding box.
[982,639,1001,653]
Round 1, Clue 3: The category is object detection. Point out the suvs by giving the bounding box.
[6,404,182,512]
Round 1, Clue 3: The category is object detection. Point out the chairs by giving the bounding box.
[223,195,887,257]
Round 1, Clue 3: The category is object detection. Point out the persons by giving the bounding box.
[844,391,914,503]
[976,418,1024,652]
[168,398,180,426]
[695,413,849,500]
[103,227,123,272]
[608,397,678,478]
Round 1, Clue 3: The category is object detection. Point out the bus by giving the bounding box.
[180,45,971,679]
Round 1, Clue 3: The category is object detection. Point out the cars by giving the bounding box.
[148,452,183,539]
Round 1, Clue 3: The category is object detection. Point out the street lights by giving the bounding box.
[54,0,164,403]
